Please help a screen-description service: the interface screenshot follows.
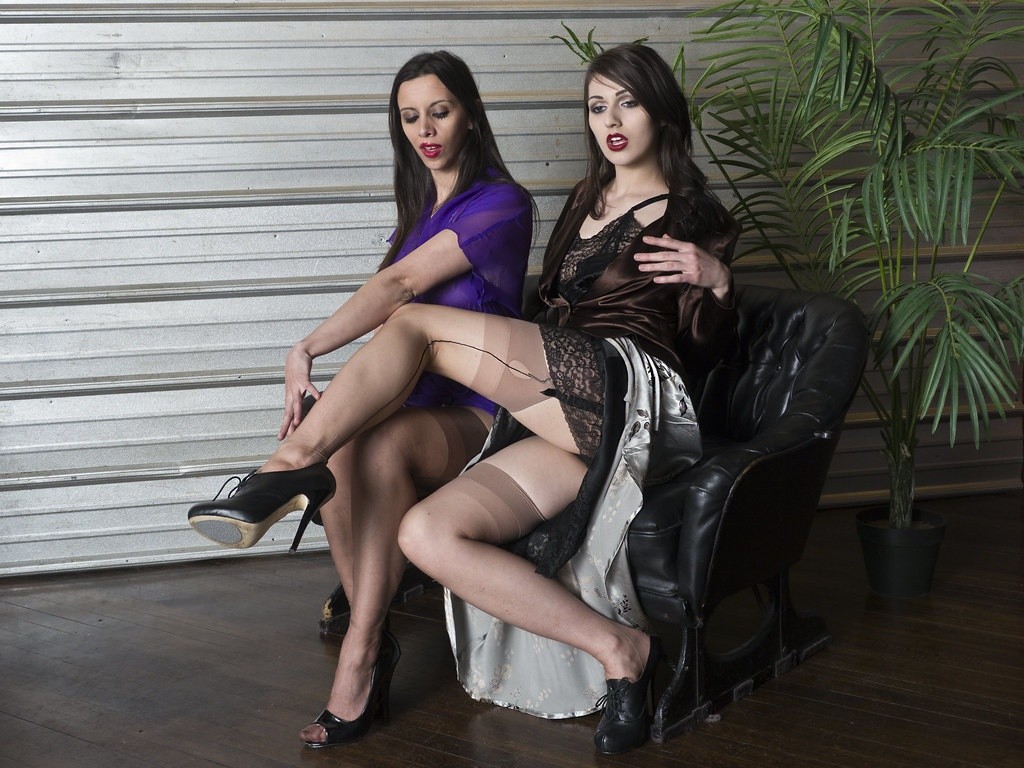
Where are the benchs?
[302,274,868,744]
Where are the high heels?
[300,630,401,749]
[594,634,663,754]
[188,463,337,555]
[317,582,350,644]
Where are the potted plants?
[548,0,1024,609]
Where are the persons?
[277,51,543,749]
[188,45,742,754]
